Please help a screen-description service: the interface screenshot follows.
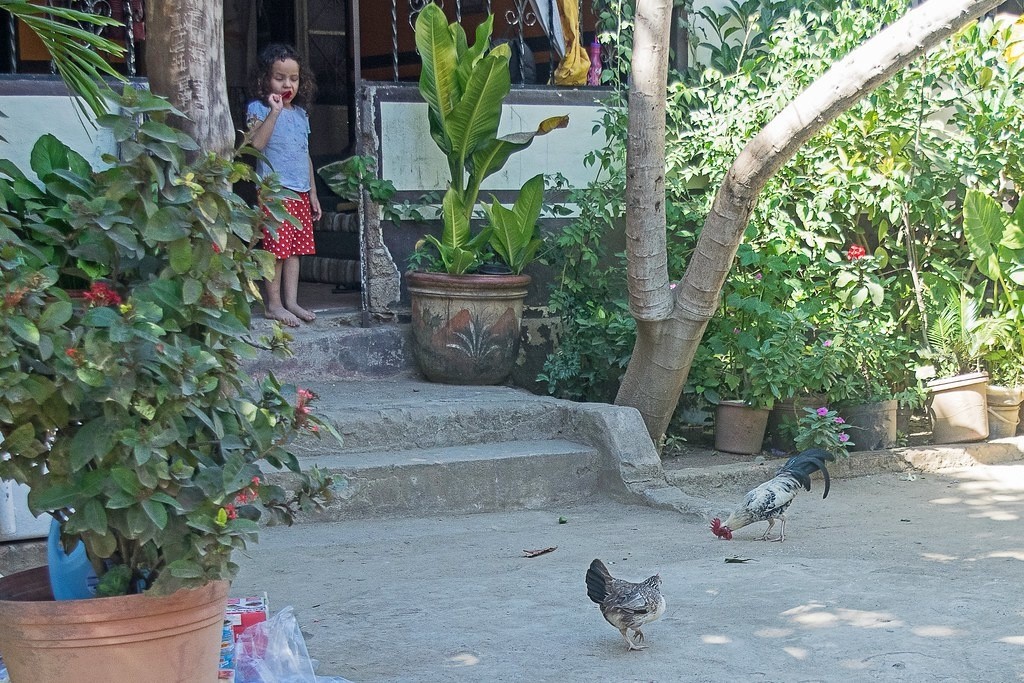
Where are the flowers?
[0,82,347,597]
[665,224,931,463]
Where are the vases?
[836,398,898,454]
[0,563,229,683]
[712,402,774,455]
[768,396,829,451]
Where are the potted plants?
[829,17,1024,443]
[407,0,571,386]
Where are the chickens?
[709,448,834,543]
[585,559,666,651]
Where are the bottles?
[220,619,237,683]
[587,42,602,86]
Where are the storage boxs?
[226,591,270,663]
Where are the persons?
[245,43,322,325]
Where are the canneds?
[218,618,239,680]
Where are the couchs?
[304,155,360,286]
